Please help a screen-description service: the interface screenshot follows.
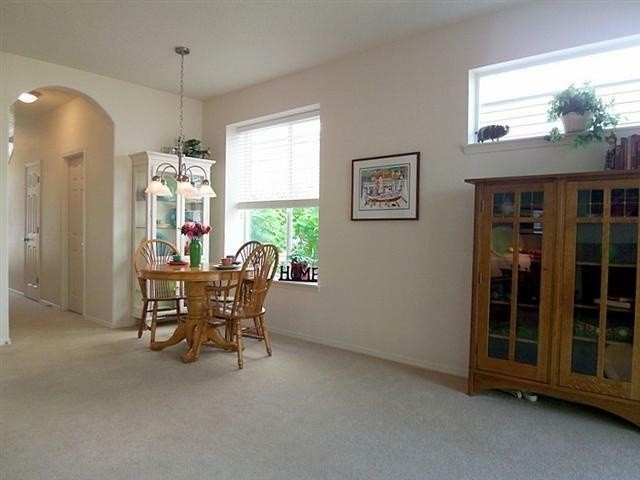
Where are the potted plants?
[544,76,624,149]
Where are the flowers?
[181,221,213,241]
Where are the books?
[593,296,632,310]
[607,295,633,302]
[614,134,640,172]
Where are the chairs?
[133,238,280,369]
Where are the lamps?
[144,47,217,200]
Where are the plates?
[167,261,188,265]
[218,264,240,268]
[215,265,237,270]
[491,225,524,256]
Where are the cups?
[226,256,236,263]
[172,255,182,262]
[221,258,233,266]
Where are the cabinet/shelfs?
[465,170,639,427]
[130,152,216,329]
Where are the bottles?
[184,241,189,255]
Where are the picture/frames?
[351,151,419,221]
